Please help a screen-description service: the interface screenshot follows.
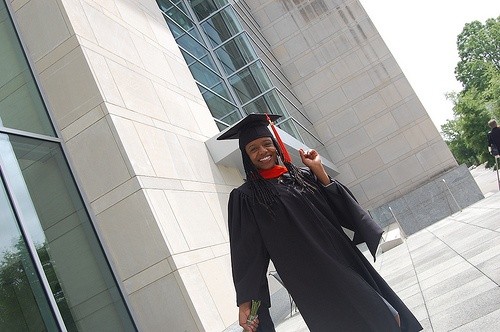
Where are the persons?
[226,122,426,332]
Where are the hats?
[216,113,283,149]
